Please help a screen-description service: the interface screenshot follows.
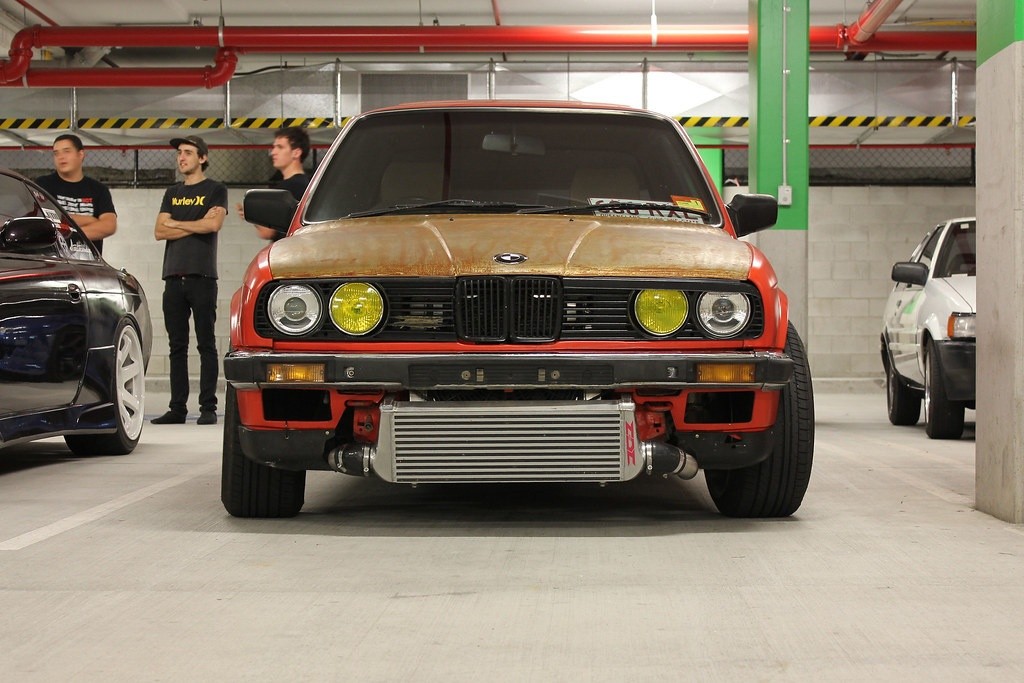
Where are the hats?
[170,135,208,155]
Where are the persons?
[237,126,310,243]
[150,136,228,424]
[34,135,117,258]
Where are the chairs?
[946,253,976,277]
[369,160,451,210]
[566,168,641,206]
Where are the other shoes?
[197,411,217,425]
[151,411,185,424]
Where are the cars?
[880,217,977,440]
[0,167,153,456]
[221,99,816,521]
